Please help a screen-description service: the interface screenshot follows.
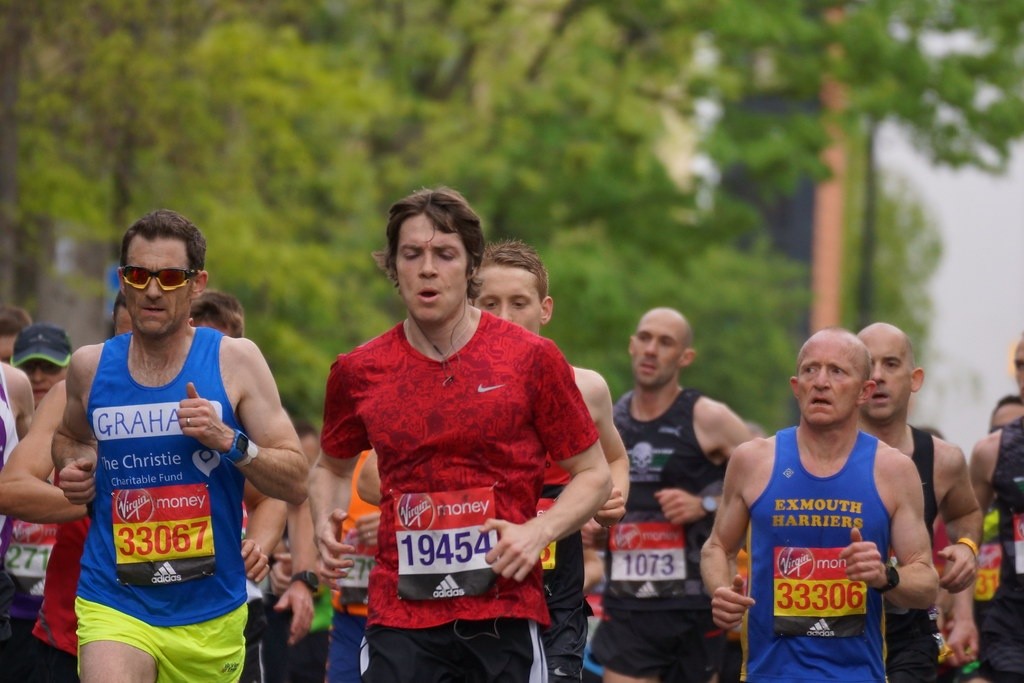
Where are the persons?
[700,328,939,682]
[310,187,614,683]
[0,291,1024,683]
[52,210,310,683]
[588,304,752,683]
[855,322,984,683]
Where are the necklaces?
[403,313,471,386]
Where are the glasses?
[122,265,196,291]
[1016,360,1023,367]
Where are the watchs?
[873,564,900,594]
[289,570,320,594]
[698,494,718,521]
[224,428,249,464]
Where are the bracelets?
[957,537,978,557]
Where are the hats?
[10,323,72,367]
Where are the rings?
[186,417,191,427]
